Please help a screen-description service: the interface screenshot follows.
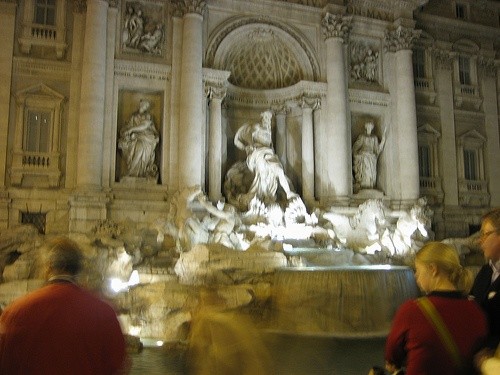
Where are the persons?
[0,188,440,297]
[117,100,161,177]
[232,111,298,199]
[349,41,382,81]
[385,360,404,375]
[468,211,500,374]
[385,242,485,375]
[122,5,172,53]
[185,307,270,375]
[0,237,130,375]
[368,366,384,375]
[352,121,388,190]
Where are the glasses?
[480,229,498,241]
